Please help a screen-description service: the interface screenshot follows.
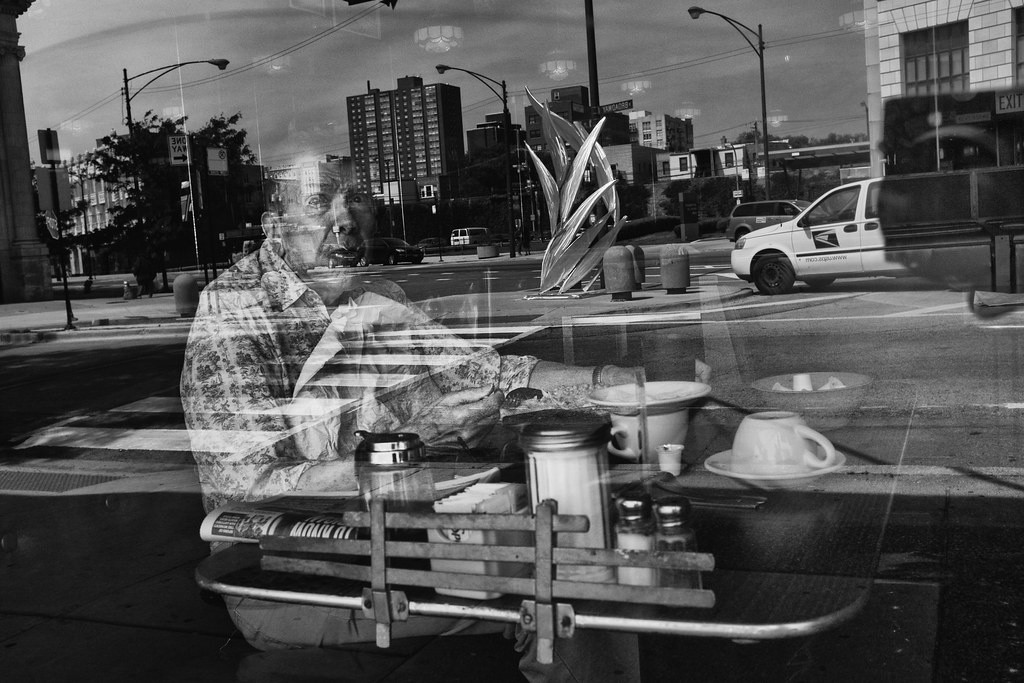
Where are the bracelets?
[590,359,608,388]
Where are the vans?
[725,198,831,243]
[450,227,490,246]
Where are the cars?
[319,244,356,269]
[357,238,424,267]
[414,237,447,250]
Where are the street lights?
[122,58,232,245]
[435,64,516,258]
[687,4,772,202]
[383,158,393,238]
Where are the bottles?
[651,495,705,596]
[614,489,656,595]
[518,415,616,592]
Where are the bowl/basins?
[750,367,880,436]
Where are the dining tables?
[193,337,922,676]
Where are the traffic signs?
[597,99,633,117]
[167,133,192,166]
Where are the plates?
[588,378,713,416]
[703,446,848,495]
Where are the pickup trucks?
[730,162,1024,296]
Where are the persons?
[183,122,657,682]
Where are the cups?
[653,443,685,479]
[455,449,499,464]
[607,413,693,465]
[729,408,835,476]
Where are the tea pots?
[349,426,441,517]
